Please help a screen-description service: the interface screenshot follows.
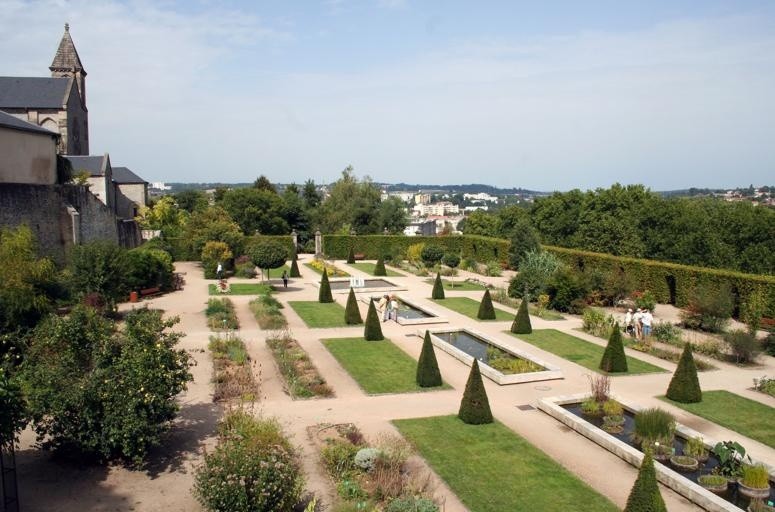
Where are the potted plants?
[736,463,771,501]
[670,456,698,472]
[697,474,728,493]
[607,404,626,426]
[711,440,752,484]
[682,435,709,463]
[607,425,624,436]
[649,444,675,461]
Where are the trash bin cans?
[130,292,137,301]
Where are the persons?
[625,308,633,336]
[639,307,654,338]
[216,262,224,281]
[281,270,290,288]
[377,293,388,323]
[632,308,642,340]
[388,294,401,322]
